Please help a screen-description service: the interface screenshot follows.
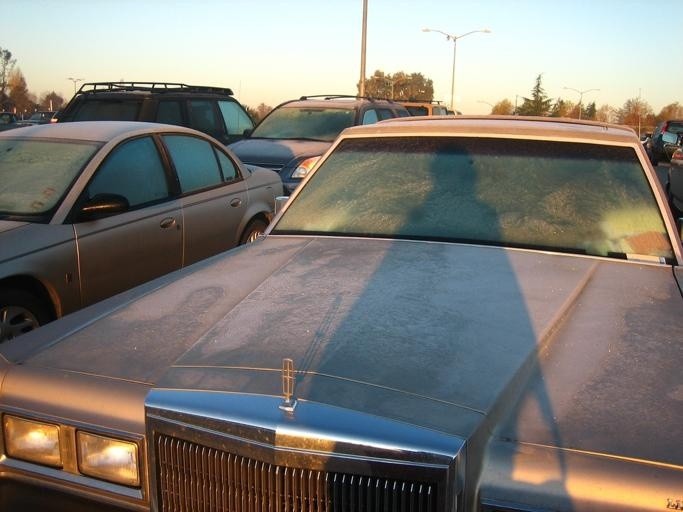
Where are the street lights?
[67,78,80,94]
[372,76,412,99]
[420,27,492,111]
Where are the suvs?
[0,114,683,512]
[392,98,447,117]
[56,82,256,144]
[225,93,411,201]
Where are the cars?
[638,119,683,222]
[446,110,463,115]
[0,120,283,346]
[0,111,62,132]
